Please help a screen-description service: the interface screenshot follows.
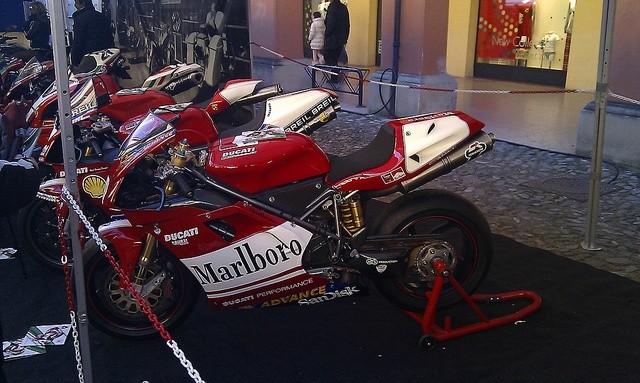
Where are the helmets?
[29,2,46,16]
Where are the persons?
[205,0,236,73]
[20,1,52,50]
[323,0,350,83]
[307,11,326,65]
[71,0,115,66]
